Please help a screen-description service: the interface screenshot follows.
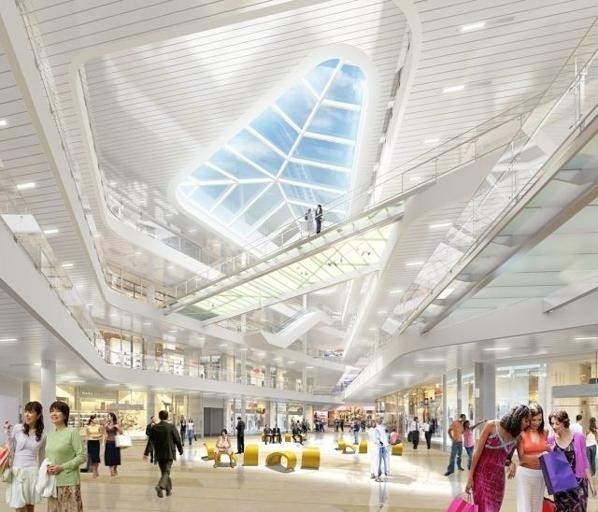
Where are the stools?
[203,434,403,469]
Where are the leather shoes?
[156,486,163,498]
[166,490,173,496]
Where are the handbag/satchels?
[447,492,479,512]
[539,448,580,493]
[586,433,597,447]
[0,438,14,482]
[114,430,133,448]
[544,497,558,511]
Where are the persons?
[4,401,47,512]
[584,417,597,478]
[314,204,323,234]
[422,417,432,450]
[512,399,552,512]
[569,415,583,435]
[545,408,588,512]
[461,419,486,471]
[303,208,315,239]
[444,414,465,477]
[44,401,87,512]
[410,416,420,450]
[103,412,123,477]
[464,405,532,512]
[142,410,400,499]
[84,415,104,478]
